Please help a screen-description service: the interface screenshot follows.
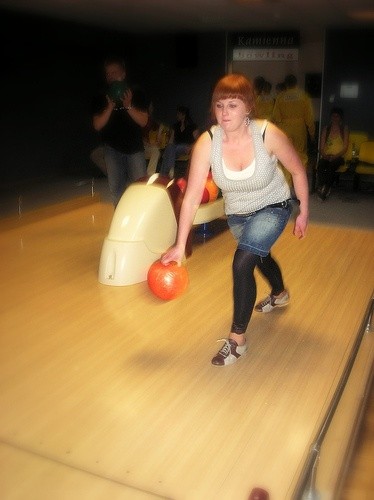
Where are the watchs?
[125,103,132,111]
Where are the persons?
[251,73,351,202]
[160,75,309,368]
[91,61,152,207]
[143,102,201,180]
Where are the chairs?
[333,131,374,193]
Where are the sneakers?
[254,289,290,312]
[211,335,248,367]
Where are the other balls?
[147,258,189,301]
[177,170,223,204]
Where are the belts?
[227,200,288,217]
